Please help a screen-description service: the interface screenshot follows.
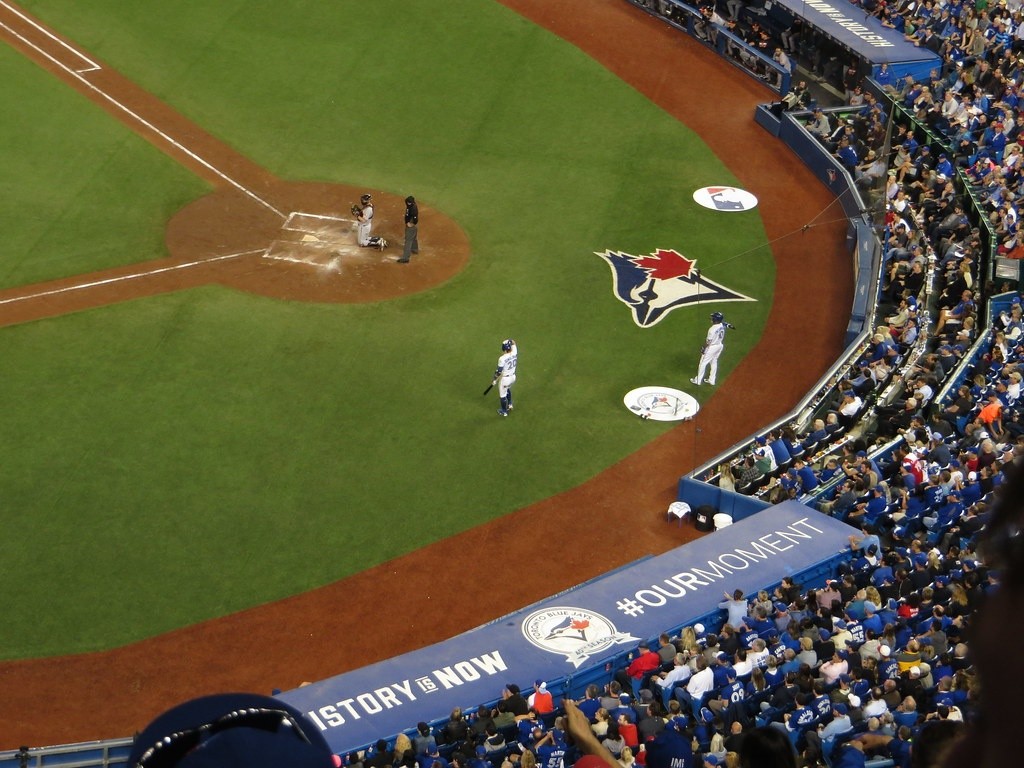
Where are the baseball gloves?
[350,204,359,217]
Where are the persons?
[492,339,518,416]
[634,0,1024,256]
[692,256,1023,522]
[351,194,389,252]
[396,196,419,263]
[332,522,1024,768]
[690,311,726,387]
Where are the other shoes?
[411,250,417,253]
[507,404,513,410]
[397,259,409,263]
[497,409,508,416]
[690,376,701,386]
[377,238,389,251]
[704,379,715,385]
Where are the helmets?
[710,311,723,322]
[361,194,371,205]
[502,340,511,351]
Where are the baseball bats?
[483,370,504,397]
[722,322,736,330]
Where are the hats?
[416,0,1024,768]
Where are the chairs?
[346,0,1024,768]
